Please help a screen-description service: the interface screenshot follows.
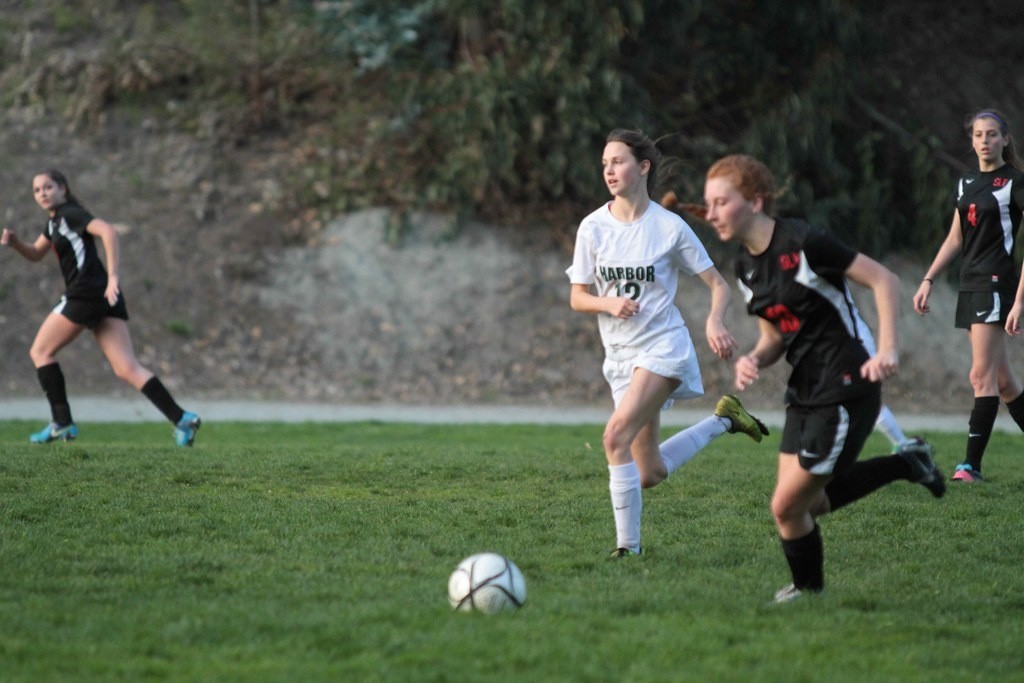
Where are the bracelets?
[922,278,934,284]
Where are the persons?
[0,170,200,448]
[565,126,771,562]
[911,109,1023,483]
[705,155,952,600]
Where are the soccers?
[445,552,528,617]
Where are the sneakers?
[891,442,903,455]
[902,436,948,499]
[174,412,200,447]
[774,582,801,602]
[607,549,644,557]
[28,422,77,442]
[951,464,982,483]
[715,394,770,443]
